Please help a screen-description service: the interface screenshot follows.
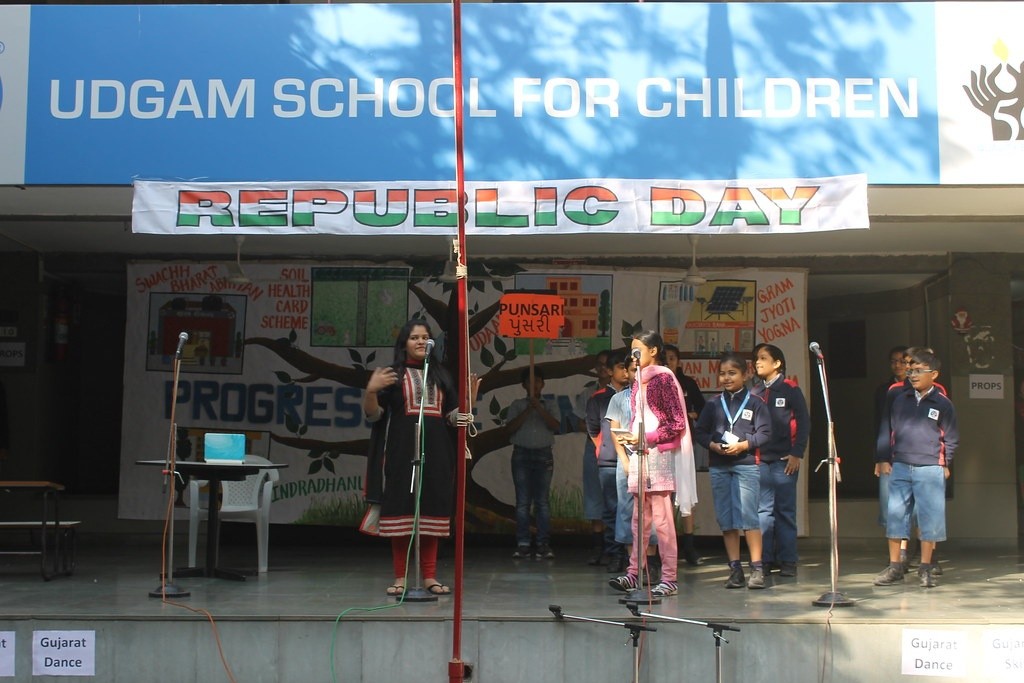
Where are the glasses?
[905,369,933,376]
[901,359,910,365]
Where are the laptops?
[205,433,245,465]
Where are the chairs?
[188,455,280,574]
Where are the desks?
[137,458,290,581]
[0,481,66,580]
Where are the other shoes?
[606,559,622,572]
[587,532,604,564]
[930,561,942,575]
[908,555,921,572]
[684,533,705,565]
[780,561,797,575]
[762,566,773,575]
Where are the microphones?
[424,338,435,359]
[176,333,188,359]
[810,342,824,360]
[632,349,642,359]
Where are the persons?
[869,346,958,586]
[574,344,706,586]
[506,370,561,559]
[610,330,688,593]
[693,355,772,592]
[362,320,483,597]
[749,344,812,576]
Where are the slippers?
[386,585,408,596]
[422,584,451,595]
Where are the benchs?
[0,520,81,578]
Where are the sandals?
[651,580,679,596]
[608,574,638,592]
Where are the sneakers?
[512,546,531,557]
[747,567,767,588]
[725,566,745,587]
[918,567,937,586]
[873,566,904,585]
[536,544,555,558]
